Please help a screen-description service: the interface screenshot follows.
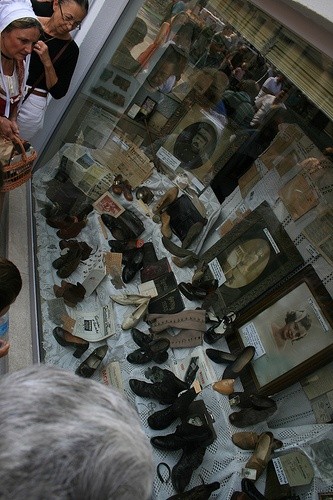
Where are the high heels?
[109,294,152,331]
[206,345,256,379]
[53,326,109,379]
[172,255,209,282]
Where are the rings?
[38,45,41,49]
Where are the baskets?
[0,135,38,193]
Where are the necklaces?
[44,18,60,47]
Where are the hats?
[197,128,211,142]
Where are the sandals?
[195,307,237,344]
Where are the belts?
[25,86,47,97]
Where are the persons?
[0,0,45,217]
[13,0,89,142]
[59,0,333,490]
[0,259,156,500]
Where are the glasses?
[60,4,81,31]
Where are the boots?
[147,307,207,350]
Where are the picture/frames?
[153,87,252,199]
[222,264,333,398]
[196,201,305,322]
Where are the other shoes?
[101,213,145,284]
[46,213,87,239]
[53,239,93,278]
[171,440,204,492]
[148,384,217,450]
[162,222,204,256]
[153,186,178,238]
[113,174,133,201]
[53,280,86,307]
[127,327,170,364]
[231,431,284,483]
[229,478,267,500]
[166,481,220,500]
[178,280,218,300]
[126,366,190,404]
[228,392,277,427]
